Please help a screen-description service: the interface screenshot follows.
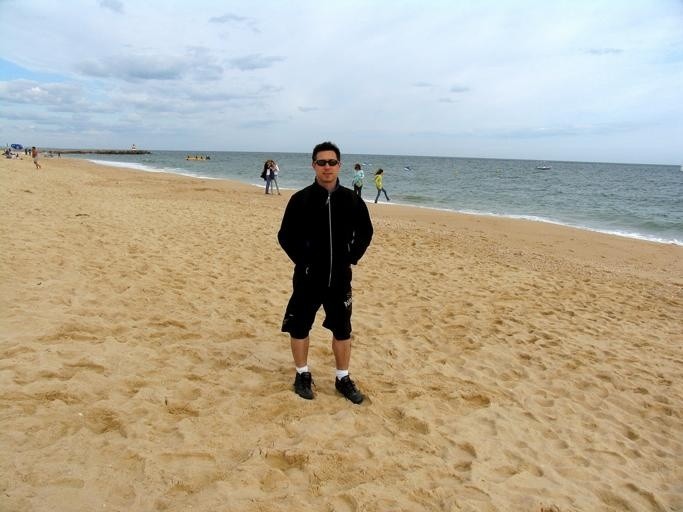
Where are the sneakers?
[335,375,363,404]
[294,371,316,399]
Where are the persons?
[262,160,282,194]
[278,142,375,403]
[373,168,390,204]
[351,163,364,198]
[3,143,58,169]
[186,154,210,161]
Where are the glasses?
[314,159,338,166]
